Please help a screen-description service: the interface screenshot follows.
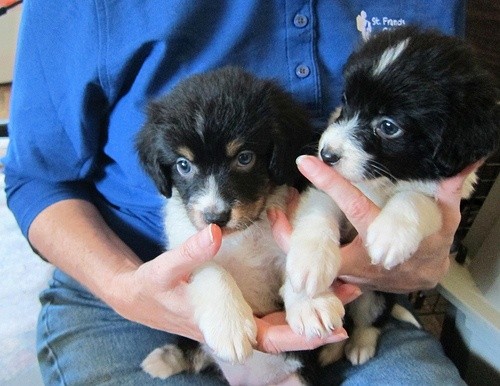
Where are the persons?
[1,1,474,386]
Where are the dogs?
[132,25,500,386]
